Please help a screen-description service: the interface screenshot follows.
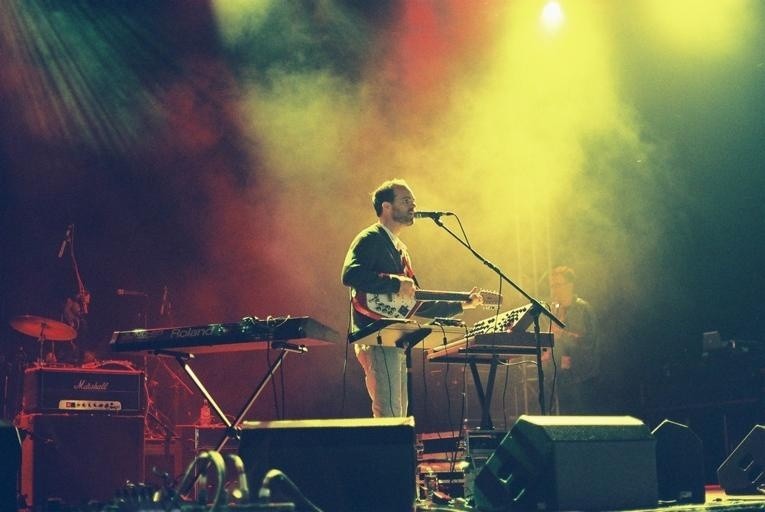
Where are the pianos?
[426,303,554,365]
[110,315,338,358]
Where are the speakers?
[234,414,417,512]
[651,419,711,502]
[473,414,659,512]
[717,424,765,494]
[19,412,147,509]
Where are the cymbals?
[9,315,78,341]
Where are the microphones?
[160,289,168,316]
[57,222,72,258]
[116,288,146,297]
[413,211,449,218]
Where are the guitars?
[351,273,503,321]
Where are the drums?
[81,360,154,422]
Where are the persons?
[341,176,486,419]
[542,265,596,416]
